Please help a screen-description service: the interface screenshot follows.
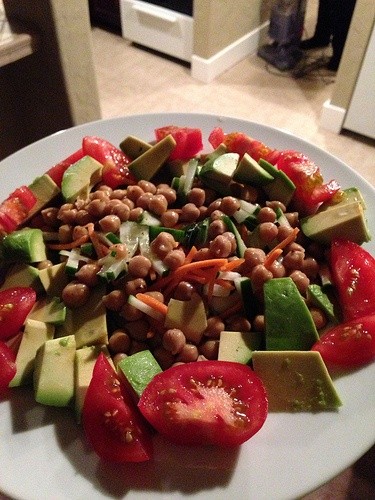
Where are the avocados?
[61,155,103,202]
[217,276,344,411]
[299,201,370,247]
[24,173,61,221]
[3,263,162,425]
[319,186,365,213]
[120,135,296,211]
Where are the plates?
[0,112,374,499]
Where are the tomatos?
[155,126,204,163]
[82,350,152,463]
[331,234,375,323]
[207,128,280,165]
[82,136,138,189]
[0,185,37,231]
[1,342,18,389]
[311,316,375,369]
[272,149,342,217]
[137,360,268,445]
[1,288,37,339]
[46,149,84,186]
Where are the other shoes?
[299,35,330,48]
[258,41,303,71]
[318,56,338,71]
